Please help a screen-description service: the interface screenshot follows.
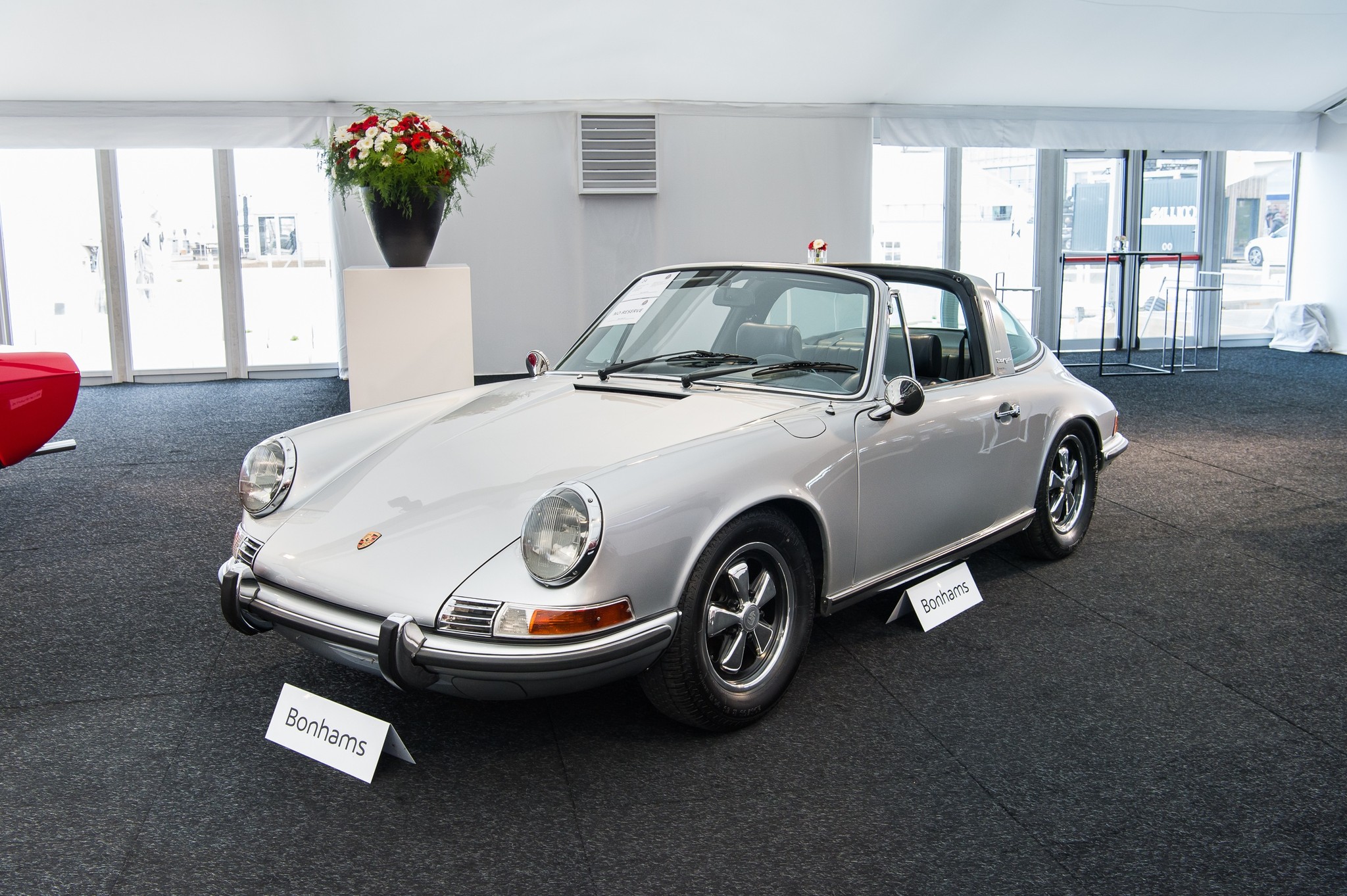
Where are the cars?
[1243,223,1289,267]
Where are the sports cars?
[212,256,1134,735]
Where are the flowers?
[303,103,495,226]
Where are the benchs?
[800,342,973,384]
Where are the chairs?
[721,323,801,367]
[840,331,945,398]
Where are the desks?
[343,263,475,413]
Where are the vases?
[359,186,445,267]
